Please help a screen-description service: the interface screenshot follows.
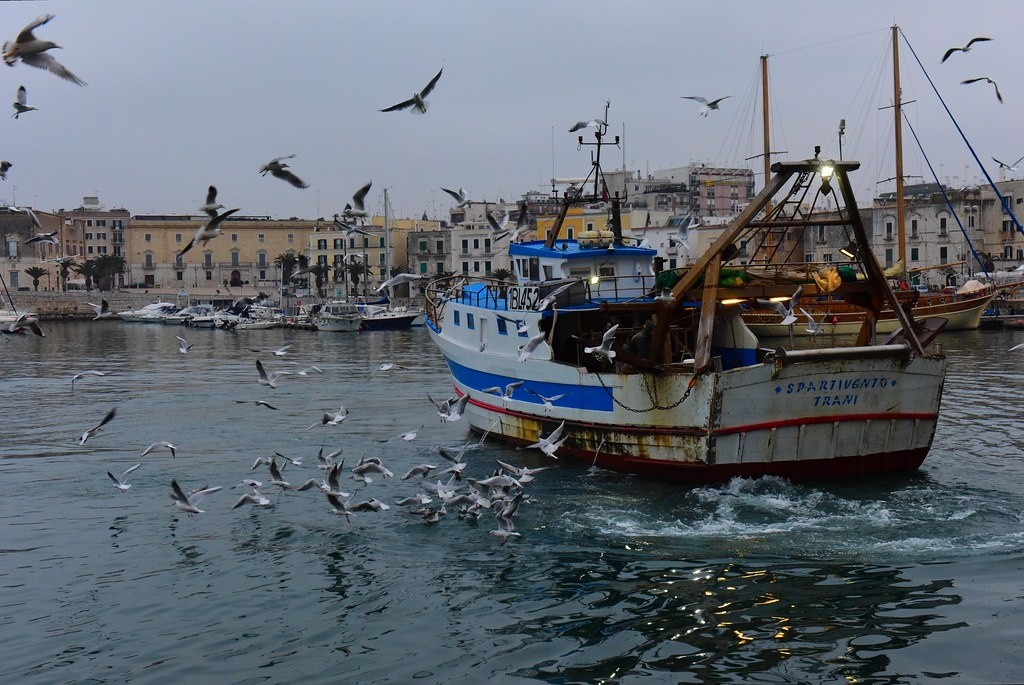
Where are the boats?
[418,145,948,489]
[0,271,40,334]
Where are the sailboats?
[113,183,432,333]
[682,19,1023,360]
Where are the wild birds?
[0,11,1024,543]
